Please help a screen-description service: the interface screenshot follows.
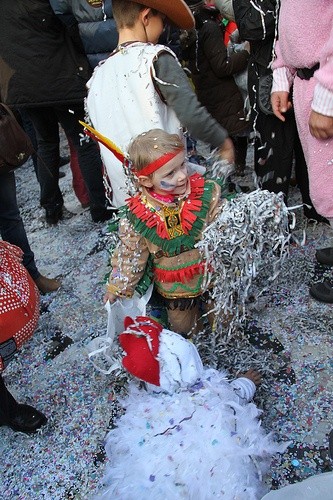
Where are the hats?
[131,0,195,31]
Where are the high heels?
[225,178,255,193]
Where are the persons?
[0,95,72,432]
[0,0,333,224]
[102,129,280,375]
[96,316,292,500]
[86,0,235,208]
[270,0,333,303]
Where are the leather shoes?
[0,404,47,431]
[35,278,61,296]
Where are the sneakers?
[309,281,333,303]
[316,248,333,267]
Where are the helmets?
[204,0,216,9]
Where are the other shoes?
[41,205,78,226]
[304,205,329,223]
[93,206,119,222]
[58,155,70,178]
